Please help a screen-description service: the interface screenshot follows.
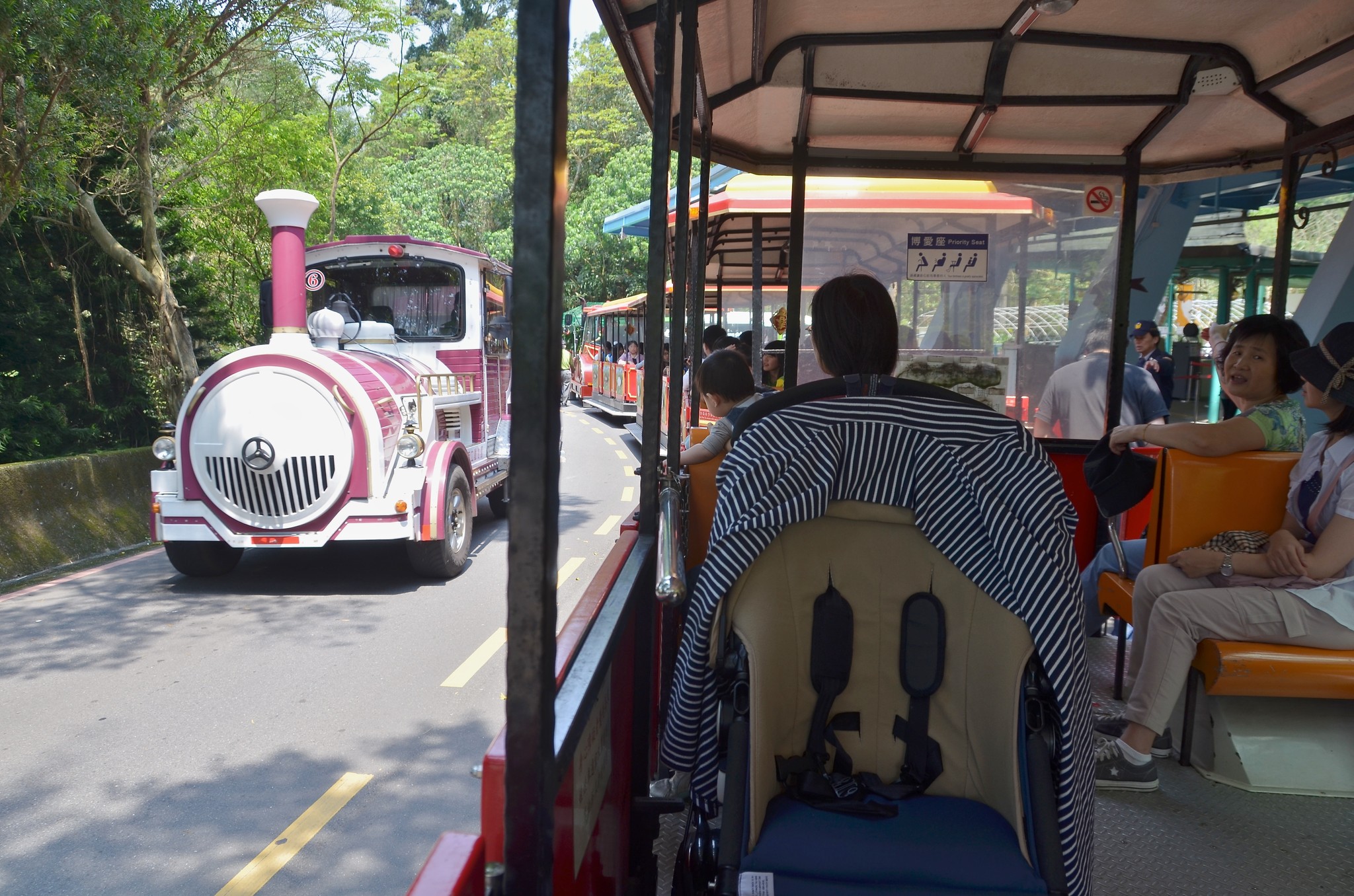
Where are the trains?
[400,1,1353,896]
[150,189,517,584]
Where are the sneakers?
[562,403,567,407]
[1092,713,1172,758]
[1093,737,1160,792]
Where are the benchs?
[1098,448,1353,768]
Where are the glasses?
[805,324,812,334]
[664,352,669,355]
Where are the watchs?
[1220,552,1235,576]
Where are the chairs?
[686,426,730,571]
[360,306,411,337]
[717,400,1068,896]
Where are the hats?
[1289,321,1354,408]
[1129,320,1158,337]
[1084,428,1157,518]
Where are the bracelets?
[1142,423,1151,445]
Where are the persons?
[439,291,461,336]
[1087,322,1354,792]
[1081,314,1310,642]
[661,349,781,475]
[706,274,898,453]
[1032,318,1170,448]
[1127,319,1174,426]
[560,324,919,407]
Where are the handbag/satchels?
[1205,539,1347,590]
